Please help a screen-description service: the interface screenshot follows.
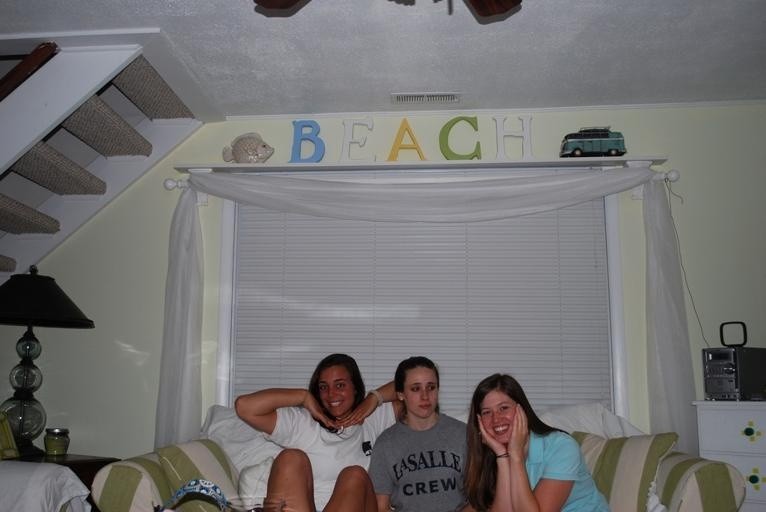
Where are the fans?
[254,1,522,18]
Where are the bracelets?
[496,452,508,458]
[368,390,384,405]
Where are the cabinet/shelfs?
[692,399,765,512]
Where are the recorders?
[702,347,766,401]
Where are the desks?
[6,454,121,512]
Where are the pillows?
[568,431,645,512]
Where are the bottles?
[44,427,70,456]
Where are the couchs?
[90,404,746,512]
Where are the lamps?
[1,268,93,455]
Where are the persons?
[233,353,405,510]
[461,371,609,512]
[366,356,477,510]
